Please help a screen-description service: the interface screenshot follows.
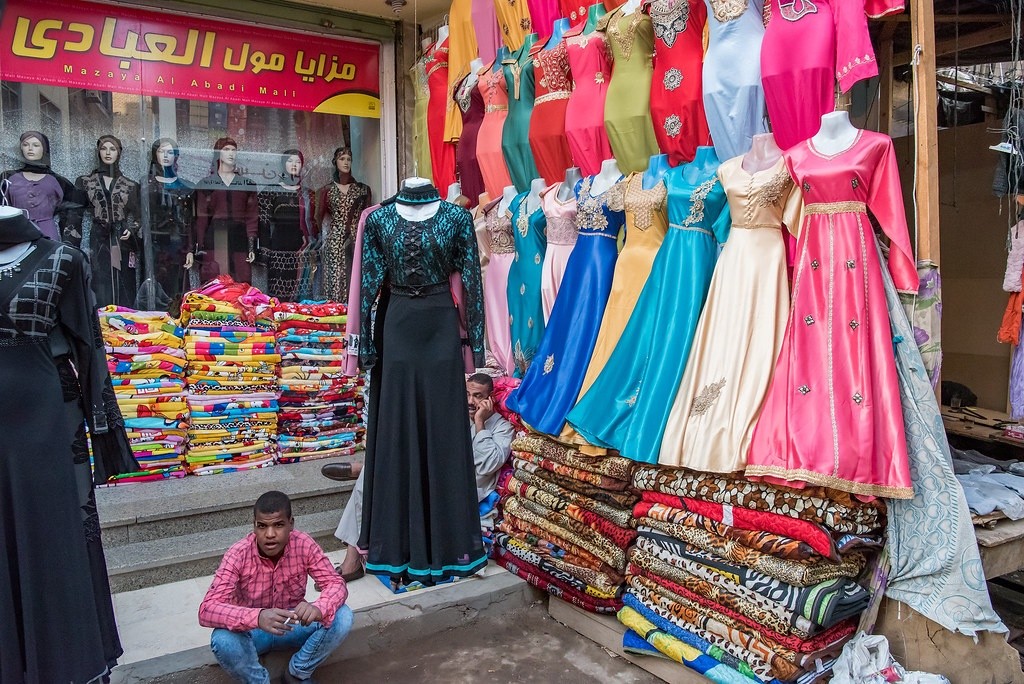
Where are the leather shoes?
[280,666,313,684]
[315,561,363,590]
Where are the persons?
[313,374,515,591]
[198,491,354,684]
[345,176,489,582]
[0,205,139,684]
[0,131,373,309]
[410,0,920,502]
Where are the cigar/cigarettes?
[283,617,291,625]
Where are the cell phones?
[485,391,497,405]
